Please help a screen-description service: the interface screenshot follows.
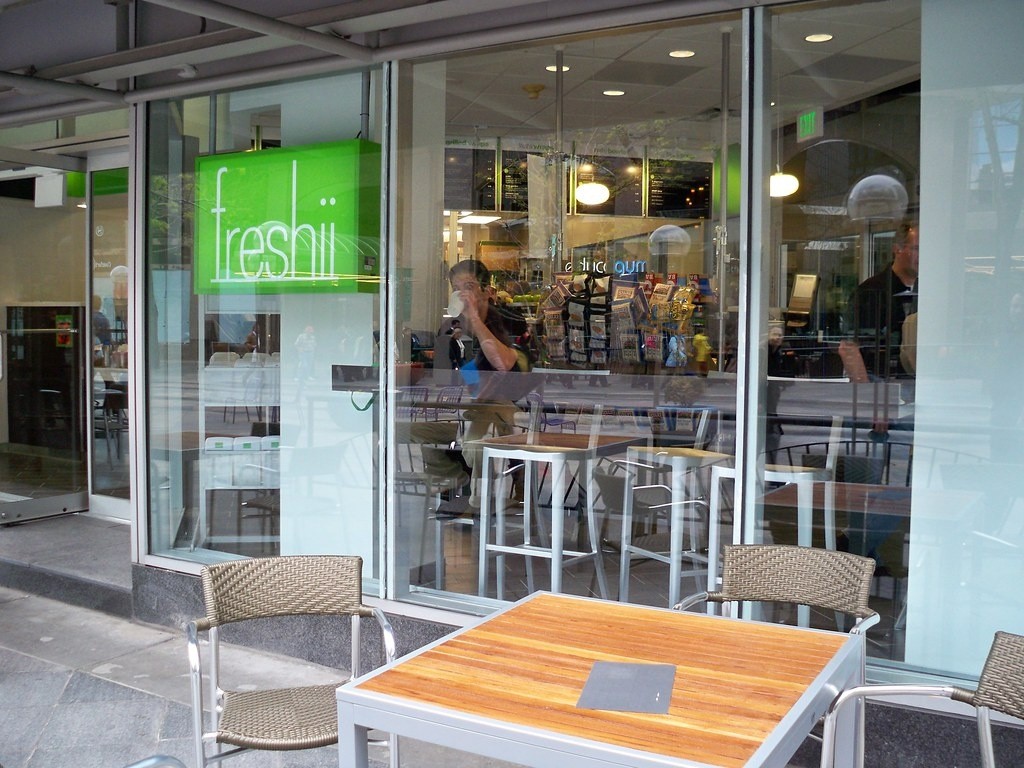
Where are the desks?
[754,480,912,635]
[334,589,867,768]
[465,431,647,600]
[334,379,914,431]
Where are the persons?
[415,259,539,518]
[838,222,920,435]
[90,295,784,473]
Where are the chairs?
[673,545,880,744]
[820,631,1024,768]
[235,491,278,557]
[187,555,401,768]
[398,369,913,665]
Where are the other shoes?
[435,495,472,519]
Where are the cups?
[447,291,465,317]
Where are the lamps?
[574,122,610,207]
[770,74,800,198]
